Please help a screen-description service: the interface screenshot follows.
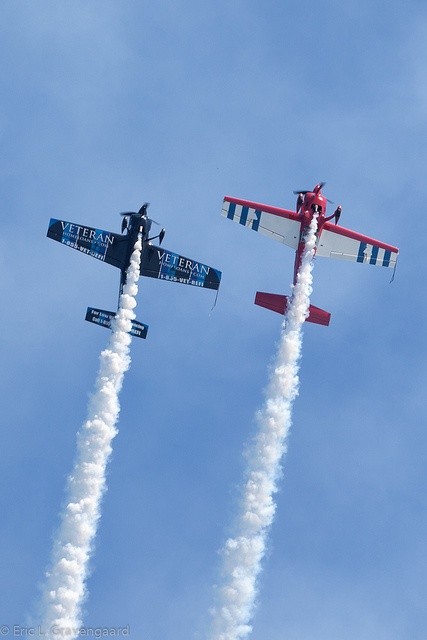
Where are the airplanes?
[46,202,222,340]
[221,183,399,326]
